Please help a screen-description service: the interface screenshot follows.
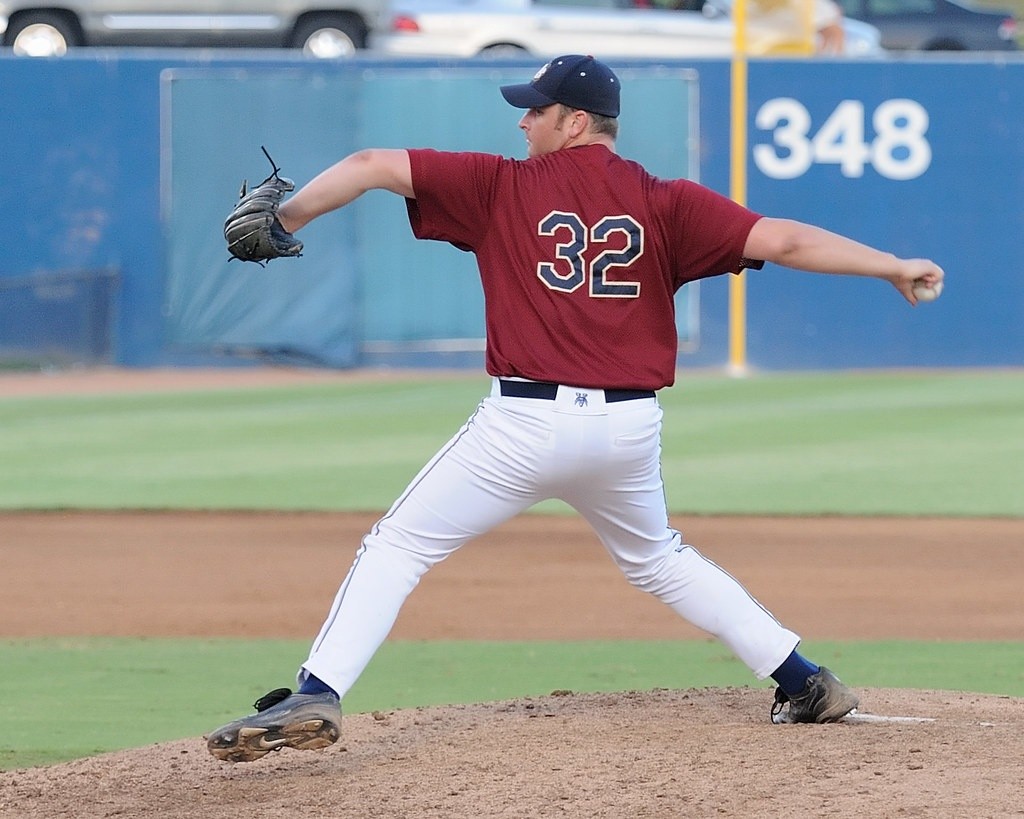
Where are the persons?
[204,55,945,763]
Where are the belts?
[498,378,656,403]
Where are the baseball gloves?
[224,144,306,266]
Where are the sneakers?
[209,687,344,763]
[769,666,863,727]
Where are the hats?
[503,57,623,120]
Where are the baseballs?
[912,276,943,303]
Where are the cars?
[840,0,1016,52]
[375,0,885,61]
[0,1,417,63]
